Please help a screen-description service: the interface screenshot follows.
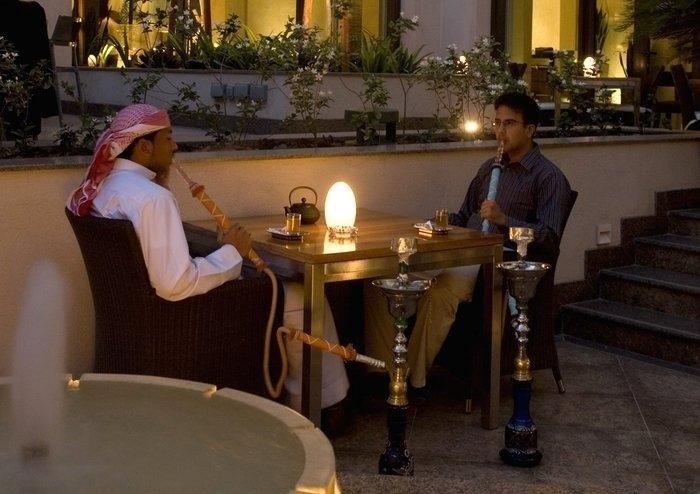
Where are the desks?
[548,75,644,132]
[182,205,506,431]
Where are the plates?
[413,223,452,235]
[266,226,313,241]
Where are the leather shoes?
[324,376,374,437]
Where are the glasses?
[494,118,529,129]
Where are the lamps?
[326,180,360,243]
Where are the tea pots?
[283,186,320,225]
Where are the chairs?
[425,188,580,416]
[670,65,700,131]
[491,63,526,84]
[60,203,281,395]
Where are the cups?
[435,208,448,230]
[286,213,301,235]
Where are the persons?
[64,102,354,432]
[364,88,569,410]
